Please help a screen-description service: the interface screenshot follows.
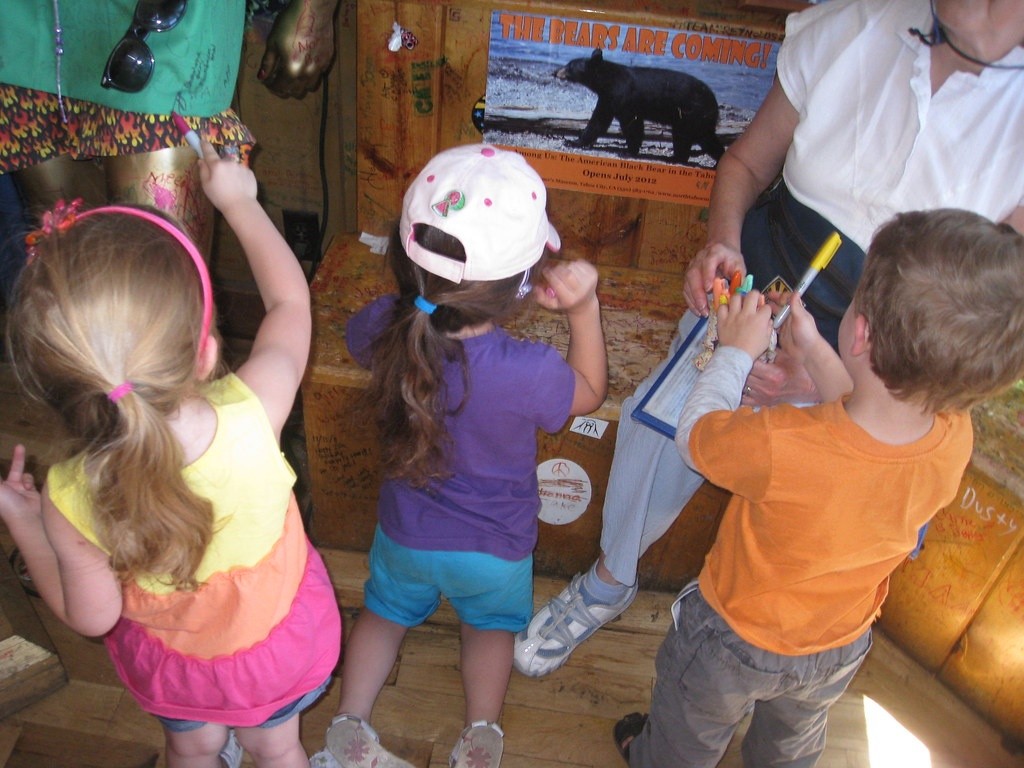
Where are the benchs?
[299,233,733,593]
[879,386,1024,747]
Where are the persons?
[0,139,342,768]
[0,1,338,266]
[512,0,1024,677]
[326,142,609,768]
[614,207,1024,768]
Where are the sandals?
[613,711,650,768]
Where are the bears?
[555,47,726,169]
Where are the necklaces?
[909,0,1024,70]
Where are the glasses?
[101,0,186,93]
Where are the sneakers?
[512,572,639,676]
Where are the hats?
[399,143,561,284]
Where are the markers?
[713,270,754,314]
[547,283,557,299]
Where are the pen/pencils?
[170,110,207,158]
[771,231,843,331]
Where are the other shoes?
[448,720,504,768]
[308,752,342,768]
[218,729,243,768]
[325,712,416,768]
